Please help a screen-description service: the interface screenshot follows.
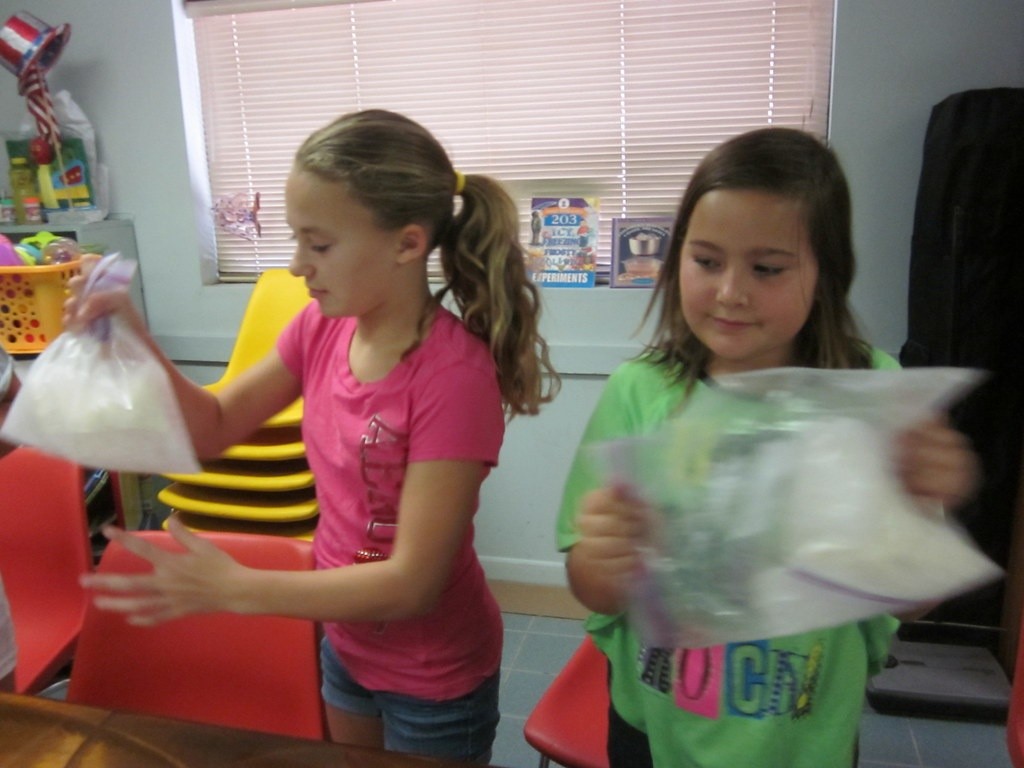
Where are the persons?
[61,108,563,768]
[554,128,975,768]
[0,349,35,692]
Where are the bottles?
[24,197,42,225]
[9,157,35,224]
[0,199,13,223]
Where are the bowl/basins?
[629,236,661,255]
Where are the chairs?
[0,269,610,768]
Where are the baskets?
[0,254,102,355]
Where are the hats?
[0,12,71,96]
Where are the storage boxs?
[7,138,93,221]
[0,253,104,355]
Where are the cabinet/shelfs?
[0,219,146,333]
